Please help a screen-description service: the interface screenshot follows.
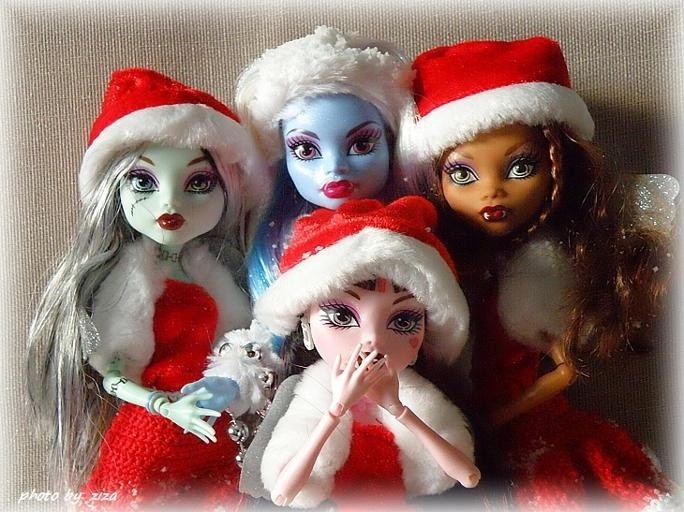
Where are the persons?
[22,25,684,512]
